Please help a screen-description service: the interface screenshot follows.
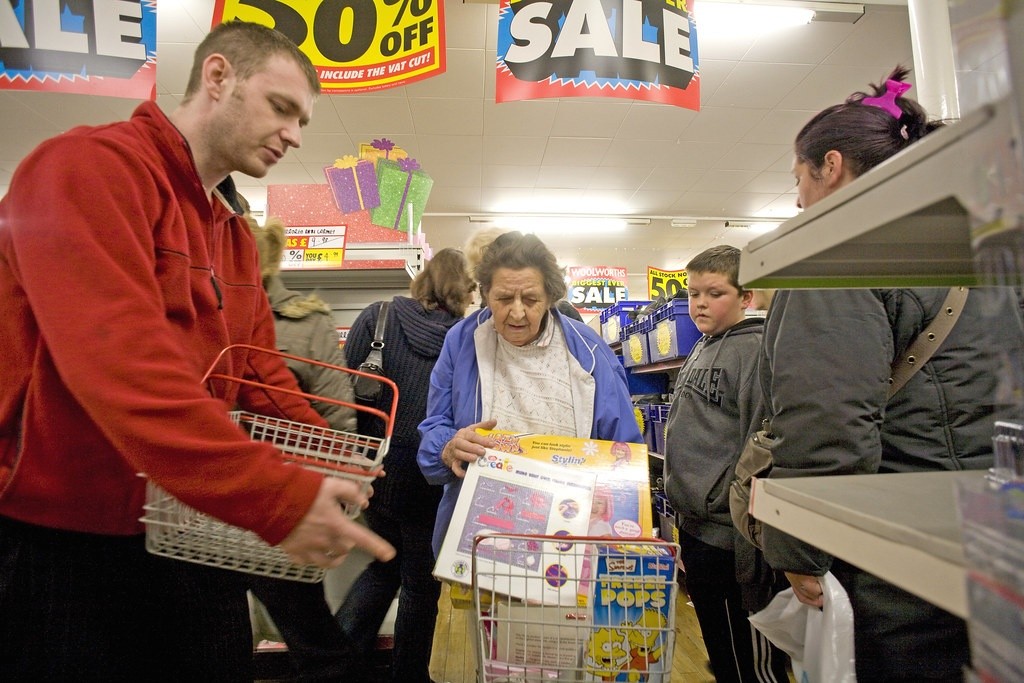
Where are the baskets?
[135,344,399,583]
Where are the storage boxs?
[586,284,710,545]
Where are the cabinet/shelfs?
[630,357,691,572]
[734,79,1024,623]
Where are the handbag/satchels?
[350,300,388,403]
[746,570,857,683]
[729,418,775,551]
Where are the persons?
[576,483,617,599]
[465,228,584,323]
[664,245,790,683]
[417,230,647,562]
[610,441,631,471]
[762,63,1024,683]
[0,20,397,683]
[334,246,476,683]
